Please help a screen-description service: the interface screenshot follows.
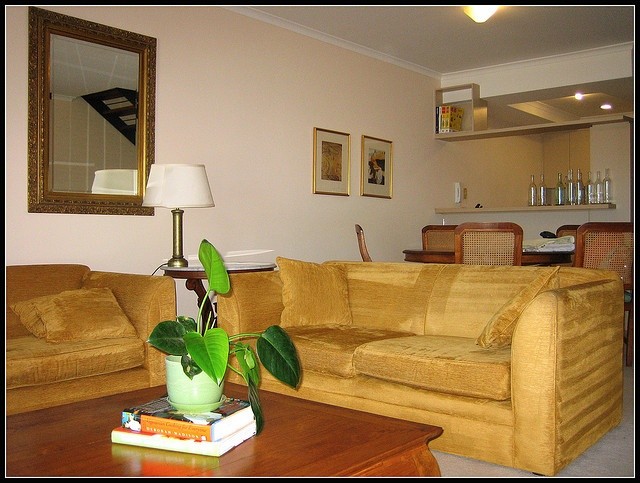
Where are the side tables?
[158,263,278,337]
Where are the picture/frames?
[312,126,352,195]
[361,132,394,198]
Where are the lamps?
[91,169,137,195]
[461,5,497,23]
[144,164,214,267]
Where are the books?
[111,419,257,457]
[121,397,254,442]
[436,105,464,134]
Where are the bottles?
[580,173,583,204]
[556,173,564,205]
[567,169,577,205]
[575,169,584,204]
[528,174,537,206]
[595,171,604,203]
[566,170,571,205]
[585,171,594,204]
[538,174,546,206]
[605,168,612,203]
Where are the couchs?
[4,265,177,414]
[210,261,623,476]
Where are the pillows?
[9,296,45,335]
[38,286,139,342]
[276,255,352,329]
[476,266,561,348]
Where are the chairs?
[454,221,522,266]
[573,221,633,366]
[354,225,374,262]
[422,224,459,252]
[555,225,580,244]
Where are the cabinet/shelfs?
[434,82,633,222]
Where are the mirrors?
[28,7,156,217]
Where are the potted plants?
[146,241,300,437]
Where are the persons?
[368,156,383,185]
